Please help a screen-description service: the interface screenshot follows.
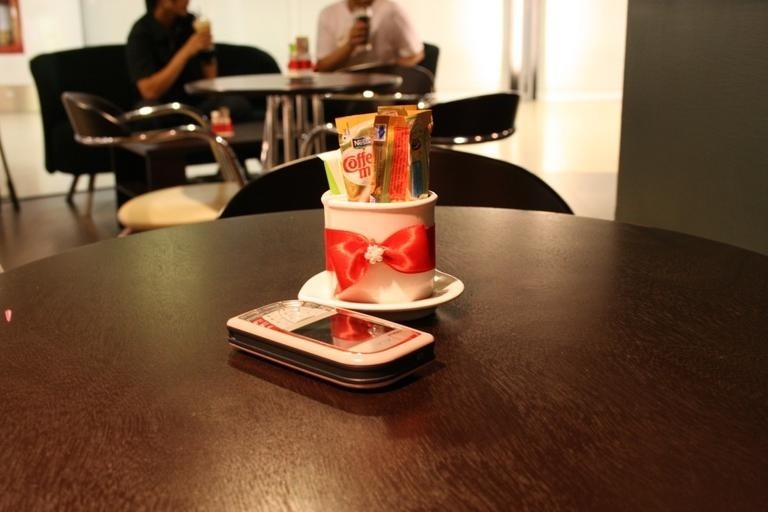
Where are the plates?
[298,267,466,322]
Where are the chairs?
[4,43,579,242]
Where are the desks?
[0,201,768,511]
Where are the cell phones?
[227,299,435,391]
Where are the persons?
[313,1,428,73]
[124,0,264,131]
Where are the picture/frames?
[0,0,25,57]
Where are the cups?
[288,53,312,79]
[210,117,235,139]
[354,15,373,52]
[194,20,209,32]
[319,188,438,303]
